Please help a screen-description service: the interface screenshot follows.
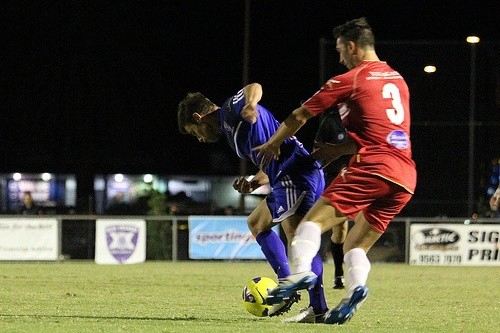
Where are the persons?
[178,83,331,324]
[21,194,43,215]
[251,19,417,325]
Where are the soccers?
[241,276,279,317]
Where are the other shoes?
[267,290,301,318]
[333,277,345,289]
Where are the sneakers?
[325,283,369,325]
[266,271,318,306]
[300,305,330,323]
